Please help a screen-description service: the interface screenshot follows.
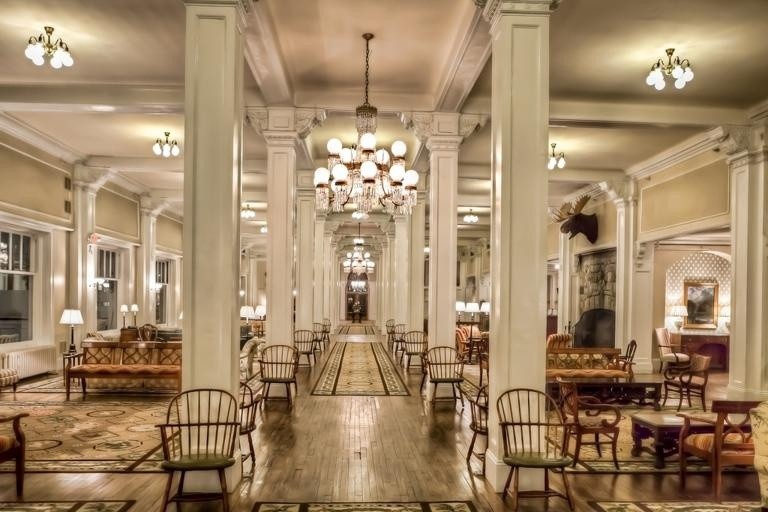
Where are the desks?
[628,411,752,470]
[63,353,83,386]
[670,333,730,373]
[547,353,609,370]
[545,373,664,411]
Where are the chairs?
[399,332,405,365]
[294,330,316,369]
[675,400,765,496]
[391,326,396,353]
[313,331,318,364]
[293,345,299,395]
[386,319,395,344]
[154,388,241,512]
[655,327,692,374]
[313,323,324,355]
[323,318,331,344]
[466,384,489,478]
[476,338,489,387]
[419,347,428,393]
[404,331,428,372]
[240,381,261,473]
[139,324,158,341]
[392,324,407,356]
[258,345,299,412]
[423,346,465,407]
[662,353,712,412]
[555,375,622,470]
[322,324,326,351]
[608,340,637,382]
[497,388,575,512]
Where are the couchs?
[546,347,636,377]
[462,326,484,358]
[151,333,182,341]
[750,402,768,509]
[547,333,572,349]
[64,342,182,401]
[85,377,178,393]
[456,328,482,363]
[239,338,266,383]
[481,332,489,353]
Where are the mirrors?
[683,282,720,329]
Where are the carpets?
[455,355,700,399]
[310,342,412,396]
[0,400,245,473]
[250,501,478,512]
[338,325,375,335]
[587,499,761,512]
[544,405,756,474]
[0,357,263,395]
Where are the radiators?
[1,345,58,379]
[103,334,120,342]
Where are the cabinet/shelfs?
[120,329,139,342]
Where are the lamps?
[255,305,266,336]
[350,273,366,289]
[120,304,129,328]
[463,209,479,223]
[240,204,256,218]
[645,48,695,91]
[313,33,419,216]
[152,132,180,157]
[178,312,183,320]
[547,143,565,169]
[342,222,375,273]
[59,309,85,352]
[669,306,688,332]
[464,302,480,364]
[352,210,370,221]
[130,304,140,327]
[25,26,74,69]
[240,305,256,338]
[260,225,267,233]
[480,301,491,332]
[456,301,465,328]
